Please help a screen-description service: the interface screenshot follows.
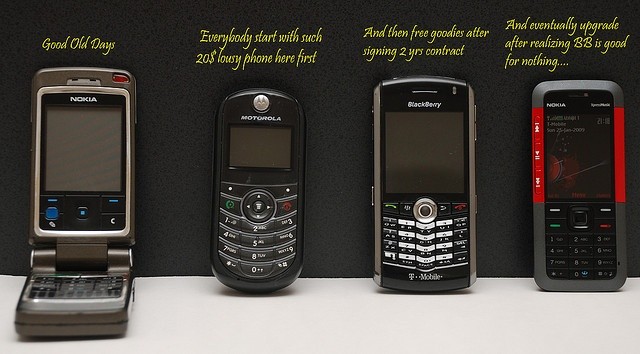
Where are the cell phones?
[530,79,627,291]
[371,71,479,291]
[14,62,137,338]
[208,85,308,291]
